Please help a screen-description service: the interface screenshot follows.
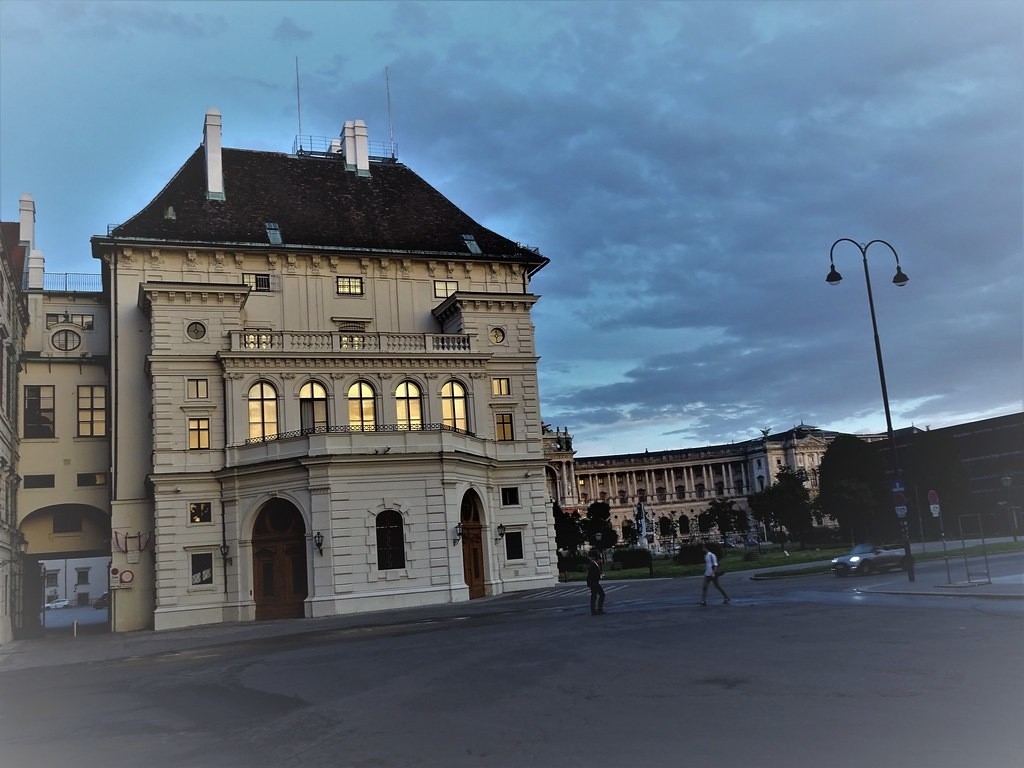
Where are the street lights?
[825,238,915,581]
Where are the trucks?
[93,592,109,611]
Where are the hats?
[595,552,602,560]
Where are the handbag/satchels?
[712,564,724,576]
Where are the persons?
[695,544,731,604]
[586,554,606,615]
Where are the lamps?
[313,531,324,557]
[220,544,233,566]
[494,524,506,545]
[453,523,465,546]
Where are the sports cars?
[829,542,907,578]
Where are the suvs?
[45,599,71,609]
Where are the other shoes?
[696,601,706,605]
[591,611,606,616]
[722,599,730,603]
